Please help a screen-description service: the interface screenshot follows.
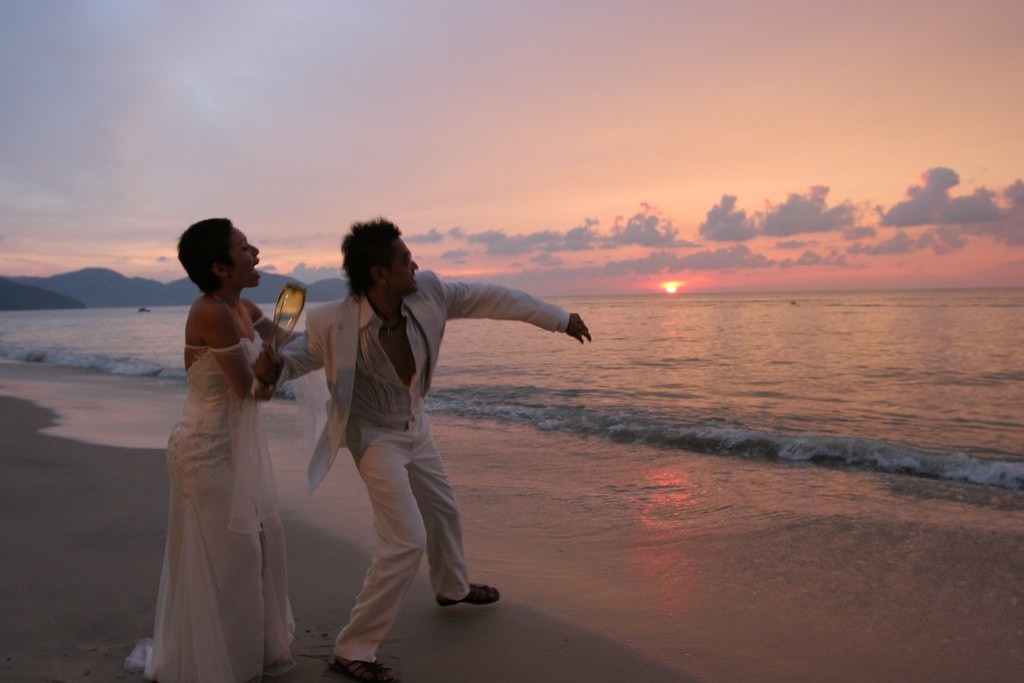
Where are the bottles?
[265,283,307,360]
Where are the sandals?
[333,655,399,683]
[436,582,500,608]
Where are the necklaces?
[379,315,401,338]
[208,290,242,316]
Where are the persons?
[165,217,308,682]
[253,218,592,682]
